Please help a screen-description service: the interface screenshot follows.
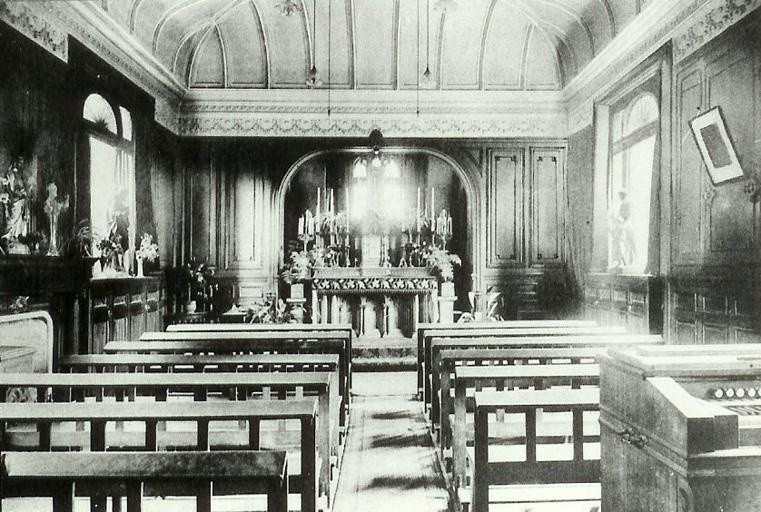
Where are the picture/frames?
[686,106,745,188]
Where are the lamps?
[421,0,435,84]
[371,146,381,167]
[306,0,323,88]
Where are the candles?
[297,185,350,235]
[400,185,454,236]
